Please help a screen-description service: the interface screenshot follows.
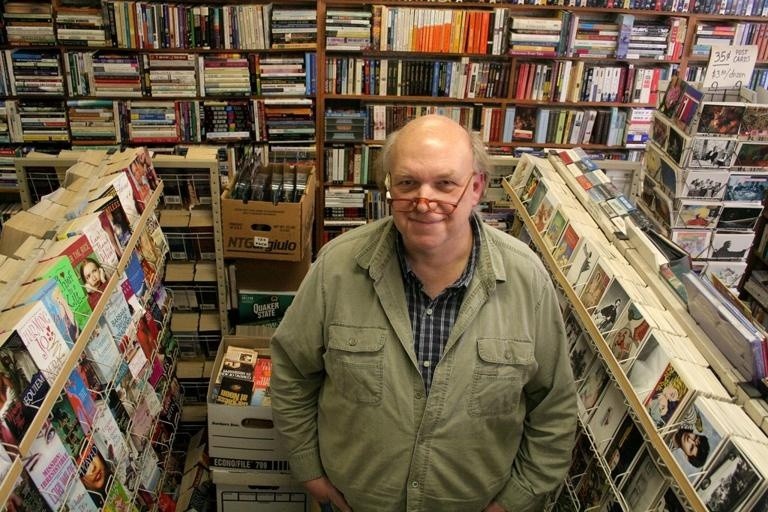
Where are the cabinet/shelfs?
[0,0,320,356]
[682,10,768,272]
[497,175,768,512]
[0,180,203,512]
[318,0,694,249]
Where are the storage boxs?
[215,483,312,512]
[220,160,315,263]
[206,337,287,463]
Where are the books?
[323,143,390,245]
[0,2,318,160]
[0,146,217,512]
[322,2,689,148]
[639,73,768,294]
[686,2,768,92]
[479,144,766,511]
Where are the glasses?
[380,167,478,220]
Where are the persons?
[269,114,578,511]
